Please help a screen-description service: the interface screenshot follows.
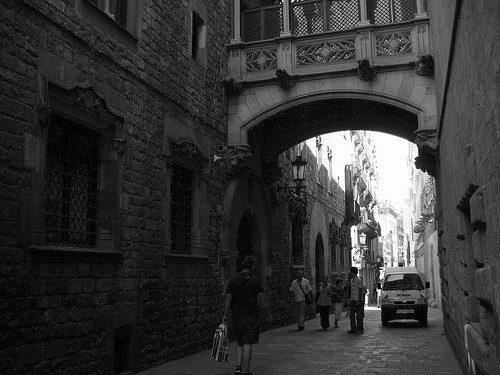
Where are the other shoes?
[347,330,363,334]
[235,365,252,375]
[298,327,304,330]
[334,323,340,327]
[323,327,327,330]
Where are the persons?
[289,270,314,330]
[223,255,272,375]
[376,282,383,308]
[317,267,367,334]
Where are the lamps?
[268,150,309,195]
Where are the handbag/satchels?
[305,292,314,306]
[211,322,231,362]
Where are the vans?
[377,267,430,327]
